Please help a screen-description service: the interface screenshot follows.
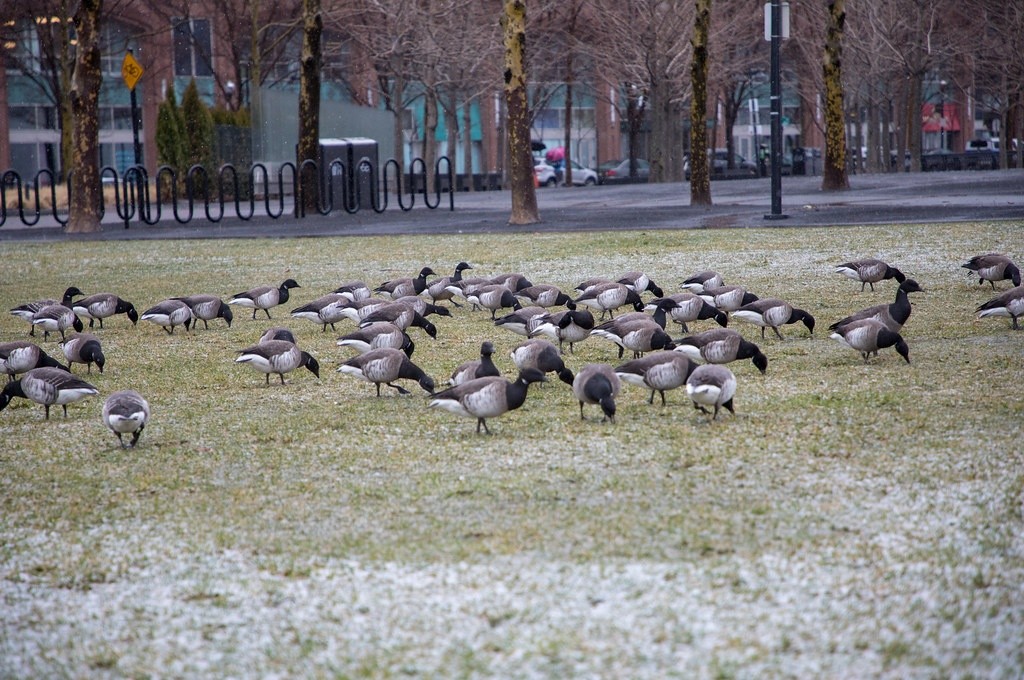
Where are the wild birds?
[423,367,550,435]
[613,351,701,406]
[491,273,533,295]
[0,367,99,419]
[57,333,106,374]
[572,364,622,424]
[651,293,728,333]
[10,286,84,337]
[466,285,522,321]
[444,279,493,312]
[258,328,296,345]
[102,389,150,448]
[670,329,768,375]
[73,293,138,329]
[169,295,233,330]
[355,303,438,339]
[617,271,663,311]
[335,324,414,360]
[493,305,550,339]
[573,277,644,321]
[518,285,576,311]
[446,341,500,387]
[30,304,83,343]
[828,319,911,365]
[419,262,474,308]
[373,266,436,300]
[227,279,301,320]
[677,272,725,295]
[335,300,391,324]
[396,296,453,318]
[686,363,737,420]
[961,253,1021,291]
[233,340,319,385]
[290,294,352,332]
[509,338,575,388]
[731,298,815,340]
[335,348,436,397]
[828,279,925,356]
[0,341,71,382]
[140,300,191,334]
[835,259,906,292]
[973,286,1024,330]
[334,281,371,302]
[695,286,760,317]
[588,296,683,359]
[530,299,594,354]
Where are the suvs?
[682,148,758,180]
[533,156,598,188]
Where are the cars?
[749,143,953,178]
[592,158,655,185]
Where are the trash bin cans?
[338,136,380,211]
[295,138,349,210]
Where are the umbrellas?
[531,140,565,162]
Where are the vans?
[964,137,1019,152]
